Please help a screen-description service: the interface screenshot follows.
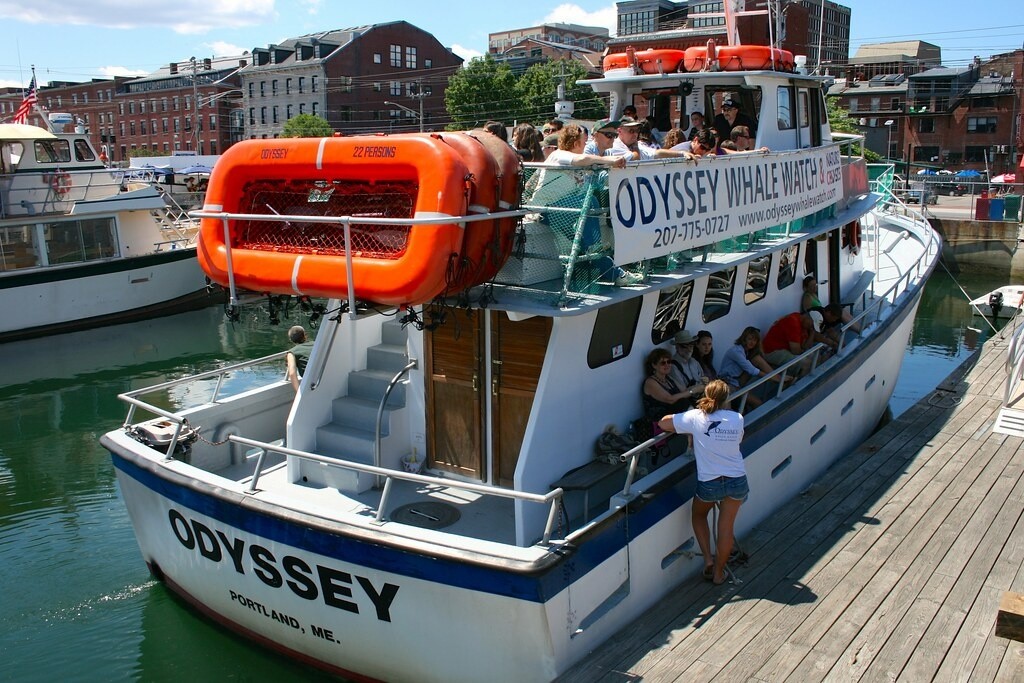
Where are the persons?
[659,380,750,584]
[481,98,771,284]
[287,326,314,391]
[644,276,861,428]
[187,177,209,201]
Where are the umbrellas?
[918,169,980,176]
[121,162,212,182]
[991,173,1015,183]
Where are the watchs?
[689,389,693,397]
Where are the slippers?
[703,566,715,579]
[713,567,728,584]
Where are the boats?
[969,283,1024,319]
[0,120,229,339]
[99,38,943,682]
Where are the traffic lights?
[1001,145,1010,154]
[990,145,999,154]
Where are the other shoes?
[782,377,797,388]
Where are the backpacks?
[633,417,672,456]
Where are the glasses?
[722,100,733,105]
[739,135,750,139]
[722,108,736,112]
[700,143,711,152]
[654,359,671,365]
[622,127,641,133]
[597,130,619,138]
[713,132,719,137]
[543,128,556,133]
[677,343,695,348]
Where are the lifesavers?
[849,221,862,255]
[52,169,72,193]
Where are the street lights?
[383,101,424,132]
[884,119,893,164]
[194,89,247,156]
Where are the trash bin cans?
[1004,195,1022,222]
[975,198,989,220]
[989,198,1004,220]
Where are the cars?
[887,174,918,189]
[935,182,968,196]
[904,187,938,204]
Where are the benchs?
[481,188,616,285]
[840,271,875,316]
[550,457,627,525]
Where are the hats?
[591,118,621,134]
[670,330,699,344]
[810,310,823,332]
[619,117,642,125]
[719,99,738,108]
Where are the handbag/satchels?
[597,430,649,464]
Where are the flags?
[14,76,38,124]
